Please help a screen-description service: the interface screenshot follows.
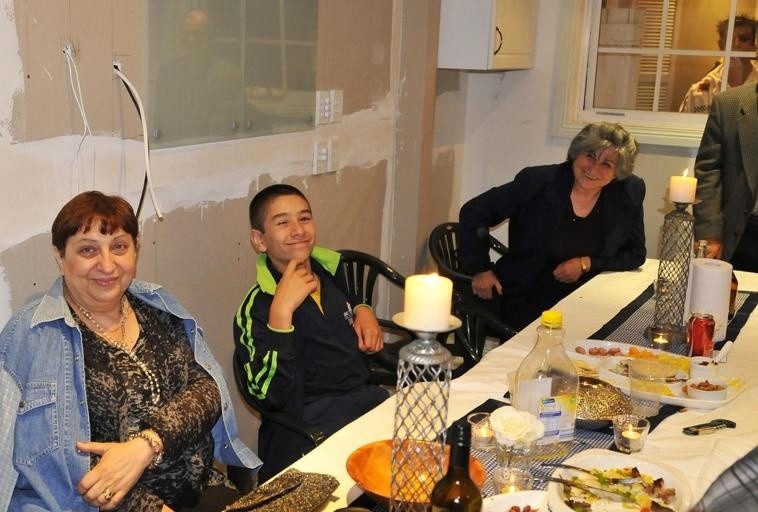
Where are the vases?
[492,468,533,494]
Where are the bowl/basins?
[343,438,484,507]
[535,373,635,431]
[687,378,729,403]
[692,355,721,381]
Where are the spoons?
[705,340,734,369]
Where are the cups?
[625,359,668,416]
[508,371,519,405]
[612,415,650,454]
[468,412,500,453]
[492,446,534,493]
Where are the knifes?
[533,476,637,506]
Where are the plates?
[547,449,691,512]
[562,337,664,372]
[477,492,567,512]
[590,357,729,412]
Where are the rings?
[103,488,114,501]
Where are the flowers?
[489,405,545,461]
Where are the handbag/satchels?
[226,468,340,512]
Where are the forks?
[541,461,643,486]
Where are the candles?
[669,171,699,205]
[404,272,453,332]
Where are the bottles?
[722,256,737,319]
[512,310,578,459]
[429,420,482,511]
[695,239,707,258]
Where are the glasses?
[731,35,751,46]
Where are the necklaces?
[69,292,161,404]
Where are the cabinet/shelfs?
[438,0,535,70]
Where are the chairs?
[428,221,523,356]
[329,248,481,392]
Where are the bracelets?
[579,257,588,274]
[126,431,164,471]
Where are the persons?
[679,13,758,114]
[458,121,646,340]
[232,184,392,476]
[691,79,757,275]
[1,190,265,511]
